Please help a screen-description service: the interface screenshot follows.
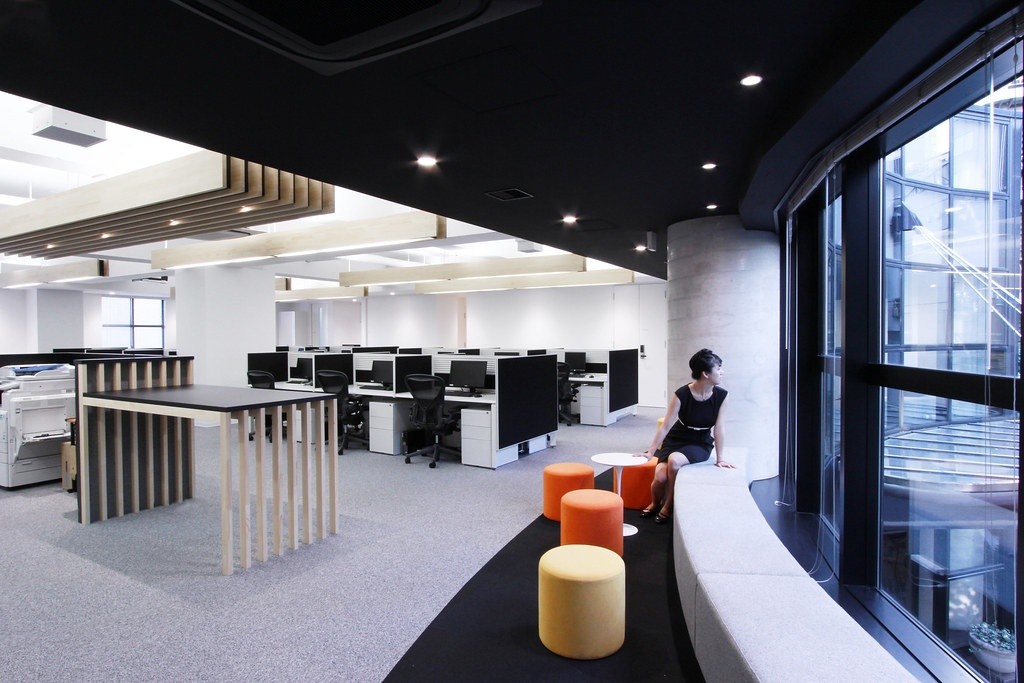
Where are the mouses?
[286,381,308,384]
[589,376,594,378]
[474,394,482,397]
[387,387,393,391]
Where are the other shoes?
[639,506,656,518]
[654,511,671,524]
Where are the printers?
[0,364,76,488]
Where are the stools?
[613,456,659,508]
[538,544,625,659]
[542,462,594,521]
[560,489,624,559]
[657,418,665,450]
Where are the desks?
[76,355,339,574]
[556,348,640,427]
[591,453,649,537]
[249,353,550,471]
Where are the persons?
[632,346,738,526]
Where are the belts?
[679,418,710,431]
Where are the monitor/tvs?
[565,352,586,374]
[295,346,547,392]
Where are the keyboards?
[359,385,387,390]
[445,390,475,397]
[569,374,585,378]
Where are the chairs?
[247,370,288,443]
[405,374,460,468]
[316,370,370,455]
[557,362,580,426]
